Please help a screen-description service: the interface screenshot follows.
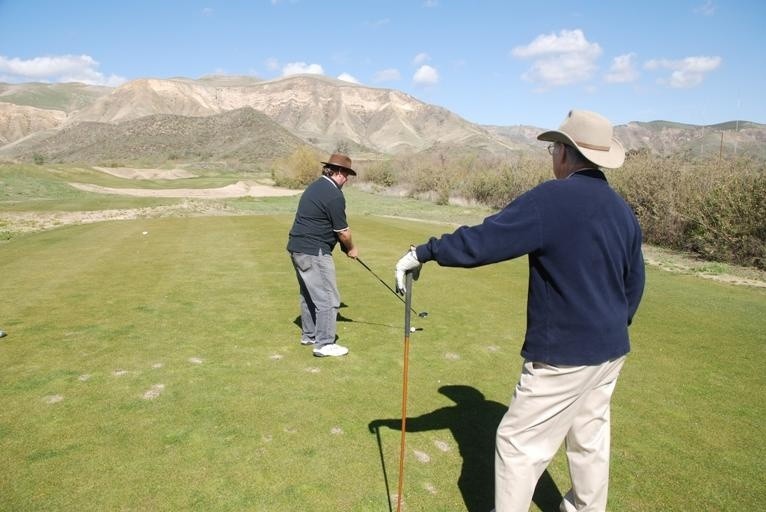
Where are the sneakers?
[312,342,349,357]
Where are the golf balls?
[410,327,416,333]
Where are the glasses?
[546,142,570,156]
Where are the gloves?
[395,244,422,296]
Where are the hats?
[536,110,625,169]
[321,153,356,176]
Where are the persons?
[391,107,649,512]
[284,153,361,358]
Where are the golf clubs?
[352,257,428,317]
